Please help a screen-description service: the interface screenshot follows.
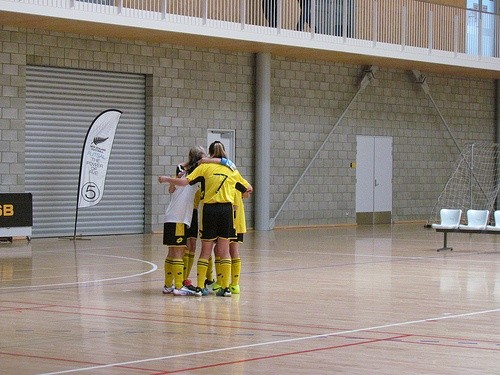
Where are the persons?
[182,145,215,290]
[210,188,246,294]
[158,141,253,297]
[163,162,201,296]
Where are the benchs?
[431,209,500,252]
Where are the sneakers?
[162,279,242,296]
